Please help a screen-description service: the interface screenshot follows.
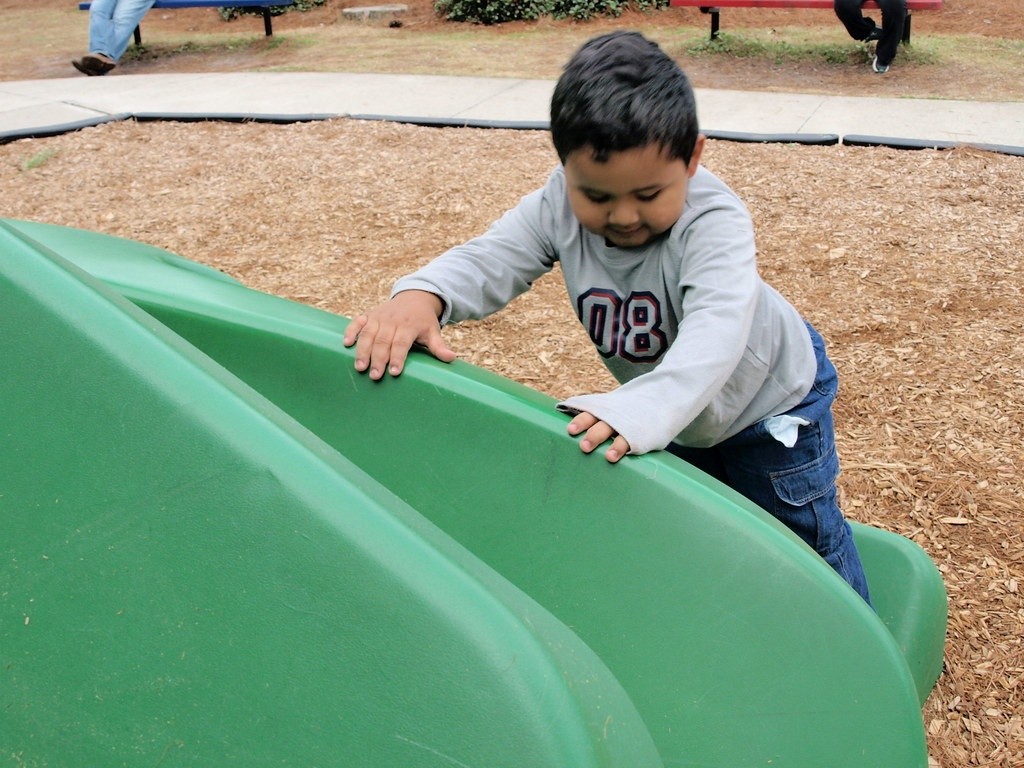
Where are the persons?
[71,0,155,77]
[343,29,876,613]
[834,0,908,73]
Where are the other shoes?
[865,23,882,43]
[872,45,889,73]
[82,54,116,74]
[72,59,105,76]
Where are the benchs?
[670,0,942,45]
[79,0,294,45]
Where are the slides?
[0,214,951,768]
